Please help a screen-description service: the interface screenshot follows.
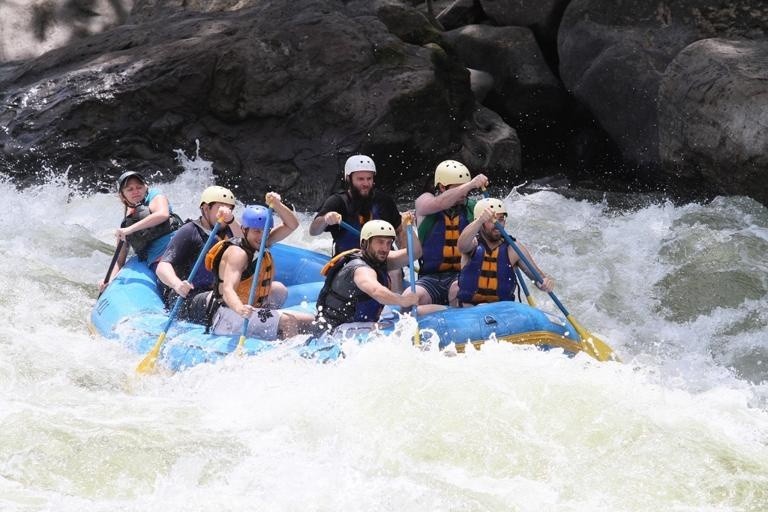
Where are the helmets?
[240,204,275,230]
[359,219,397,248]
[345,155,377,182]
[198,185,236,212]
[117,170,146,193]
[433,159,472,189]
[473,197,509,221]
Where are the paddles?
[494,220,621,364]
[137,216,229,376]
[226,203,275,368]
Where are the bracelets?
[224,217,234,225]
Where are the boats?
[91,237,620,378]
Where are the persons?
[310,155,404,292]
[210,192,313,345]
[457,198,553,306]
[315,212,423,336]
[155,183,288,323]
[98,172,184,291]
[402,159,489,315]
